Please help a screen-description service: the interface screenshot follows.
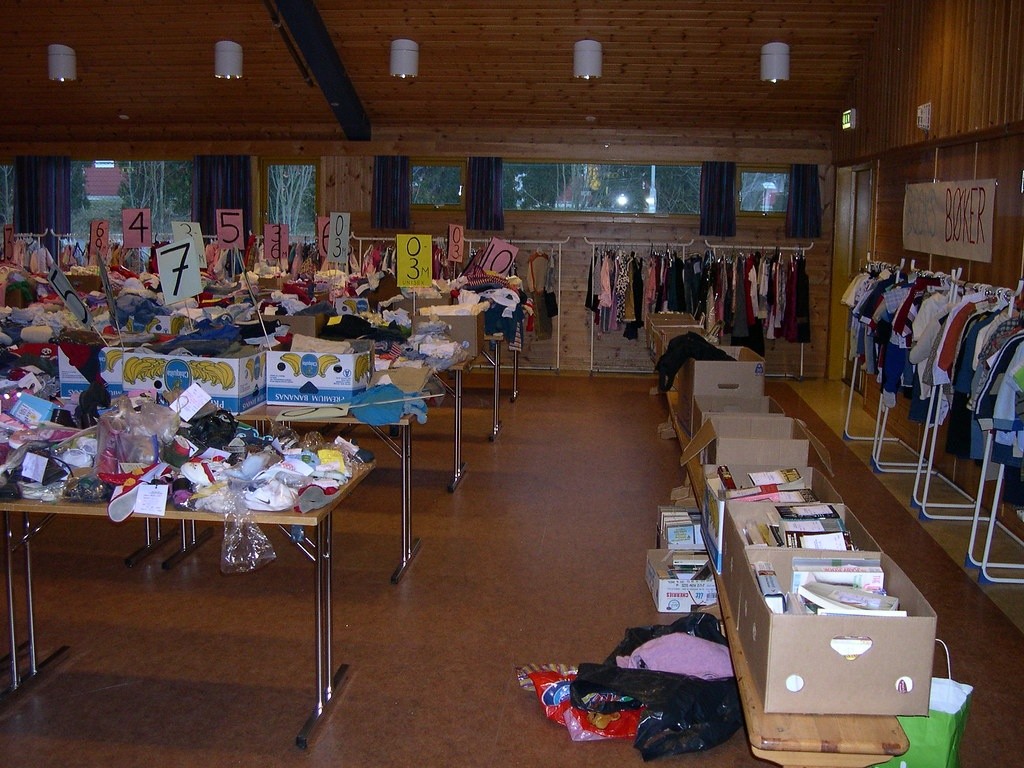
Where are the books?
[655,468,912,695]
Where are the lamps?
[759,41,790,86]
[48,45,77,83]
[572,40,602,80]
[212,40,244,81]
[390,39,419,79]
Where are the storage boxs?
[57,345,140,406]
[413,306,487,357]
[645,313,695,350]
[258,309,327,334]
[66,273,102,293]
[649,326,719,365]
[718,504,887,631]
[259,273,295,289]
[644,549,719,612]
[122,351,267,416]
[678,418,835,478]
[212,293,257,308]
[675,346,768,434]
[737,548,938,718]
[3,287,29,310]
[113,313,192,337]
[392,292,452,320]
[265,347,374,406]
[656,471,707,549]
[694,395,785,440]
[333,296,371,317]
[701,464,845,575]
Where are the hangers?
[12,232,808,276]
[858,259,1024,319]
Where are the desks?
[0,441,378,754]
[160,346,433,586]
[280,323,479,496]
[388,331,504,443]
